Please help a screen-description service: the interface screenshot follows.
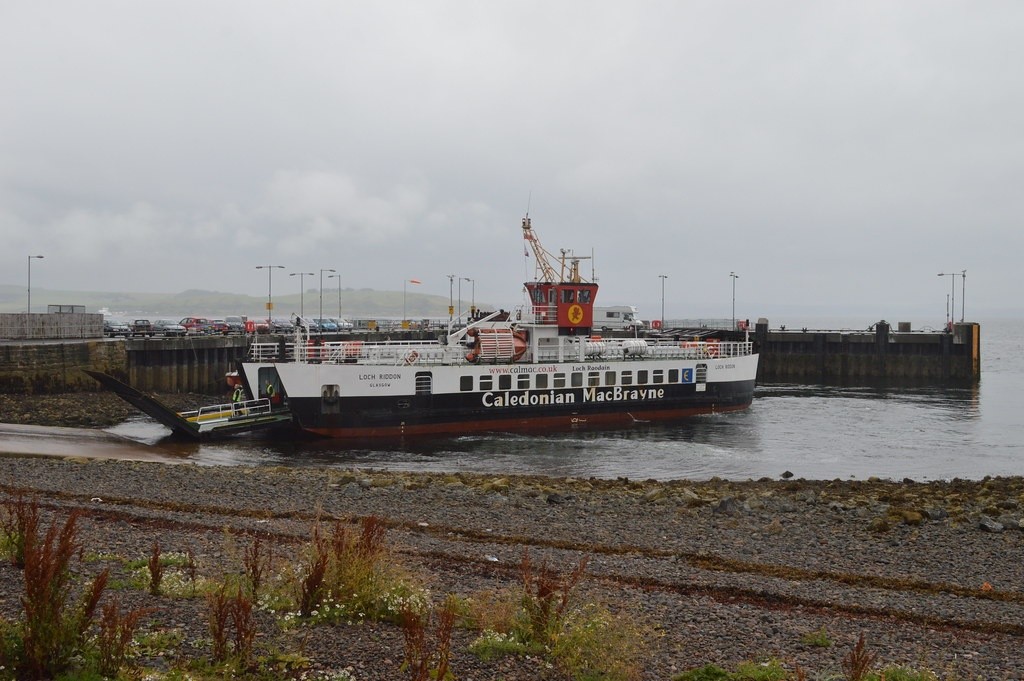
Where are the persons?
[264,380,275,412]
[232,384,243,417]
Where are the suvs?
[128,319,153,337]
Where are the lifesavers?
[404,350,418,363]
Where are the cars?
[331,318,353,331]
[270,318,294,334]
[103,319,131,338]
[313,319,339,332]
[180,316,269,337]
[300,317,317,334]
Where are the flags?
[523,242,530,257]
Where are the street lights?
[329,275,341,319]
[320,269,336,333]
[289,273,315,318]
[446,274,455,322]
[468,279,475,318]
[937,273,966,325]
[255,264,285,334]
[27,256,44,314]
[658,274,669,329]
[458,277,469,330]
[730,271,740,330]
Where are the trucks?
[591,306,643,332]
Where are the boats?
[84,188,759,446]
[463,329,527,363]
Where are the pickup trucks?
[149,320,188,337]
